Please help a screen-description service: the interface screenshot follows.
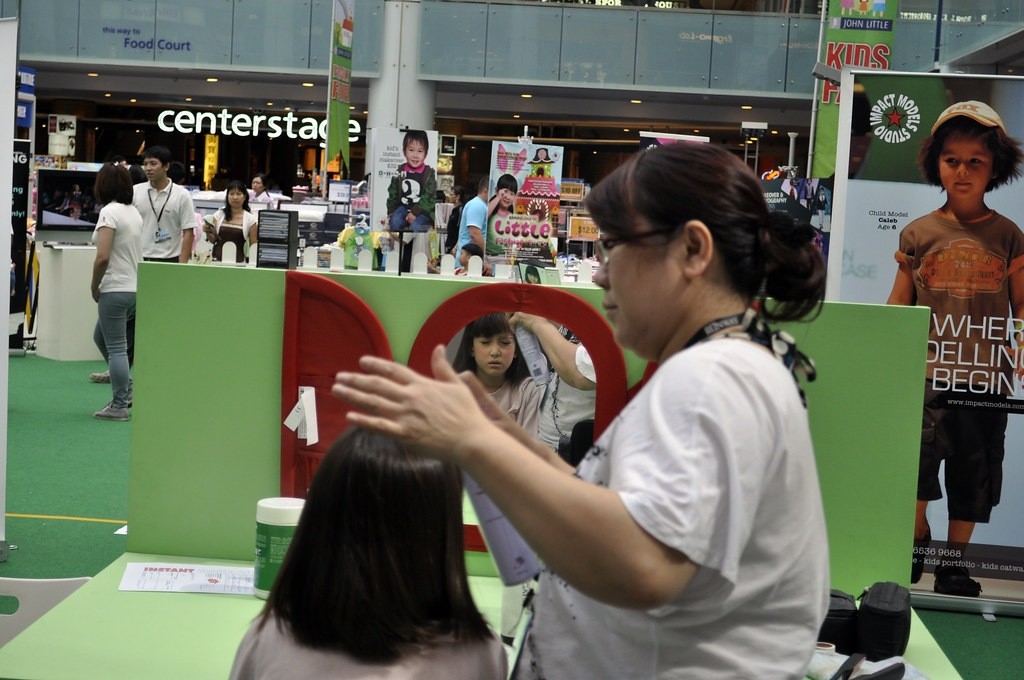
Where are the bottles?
[458,467,542,587]
[515,322,553,388]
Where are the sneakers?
[911,522,931,584]
[93,406,129,420]
[933,566,981,597]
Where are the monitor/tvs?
[36,169,111,232]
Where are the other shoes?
[90,370,111,382]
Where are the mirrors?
[407,282,626,558]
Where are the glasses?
[593,225,678,265]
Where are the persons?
[451,309,539,439]
[90,147,277,420]
[386,129,435,231]
[224,430,510,680]
[443,174,490,277]
[882,97,1024,596]
[506,311,597,455]
[332,143,833,680]
[487,173,517,219]
[525,266,541,284]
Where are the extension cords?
[25,349,37,355]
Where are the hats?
[931,100,1006,133]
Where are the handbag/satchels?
[816,588,857,659]
[856,582,912,663]
